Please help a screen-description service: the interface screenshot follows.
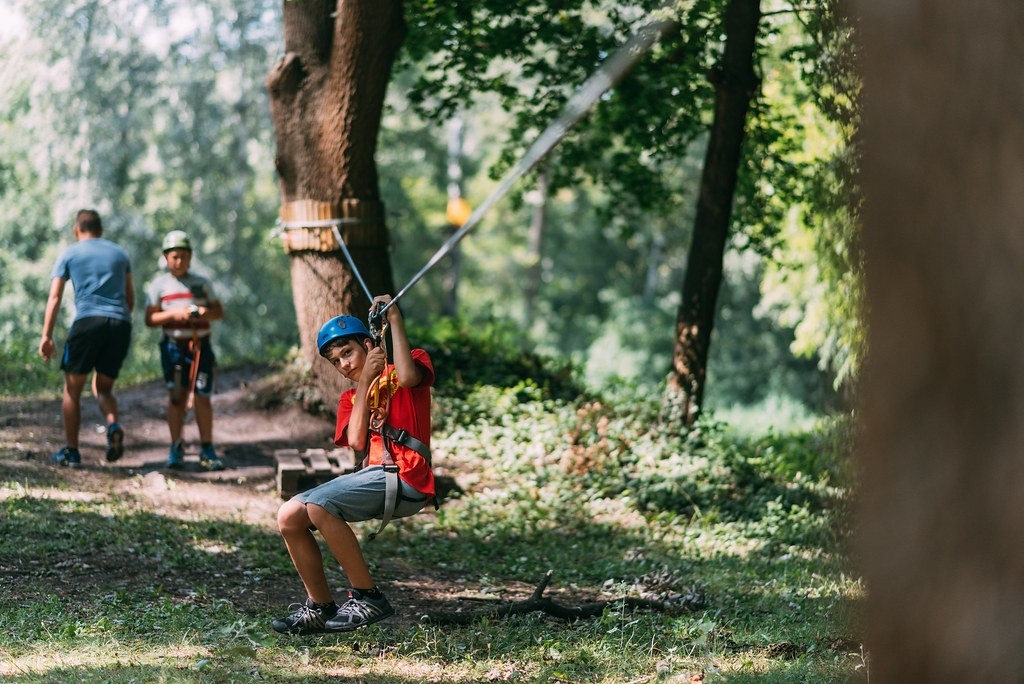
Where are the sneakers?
[271,600,339,635]
[52,446,80,468]
[198,448,223,471]
[106,423,125,462]
[168,439,186,468]
[325,589,395,631]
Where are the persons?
[39,209,134,467]
[272,294,433,634]
[145,230,223,471]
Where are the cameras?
[188,304,199,317]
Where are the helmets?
[161,230,191,255]
[316,316,368,355]
